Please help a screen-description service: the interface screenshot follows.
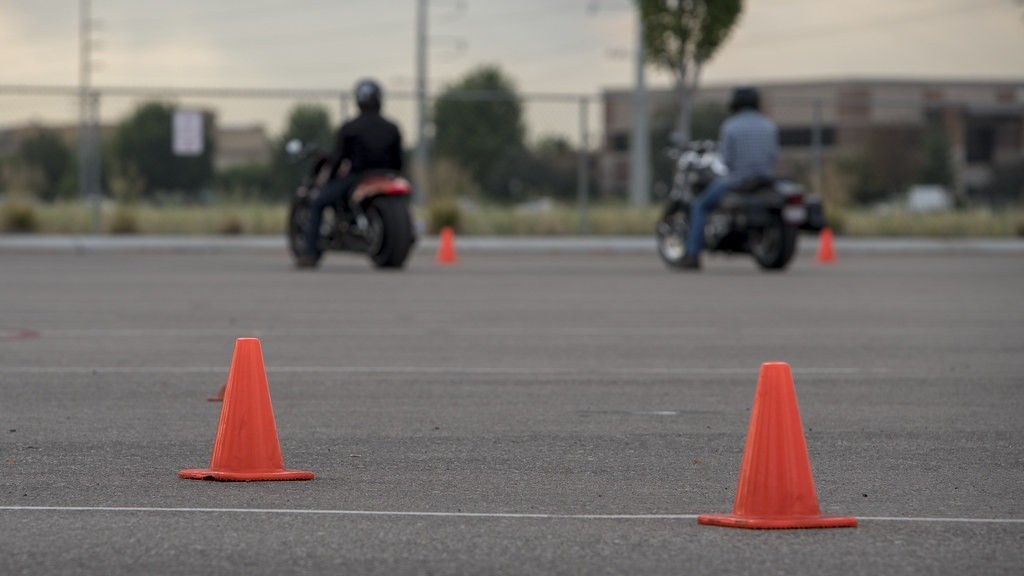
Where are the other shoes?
[674,252,699,269]
[295,253,317,267]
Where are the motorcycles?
[285,137,415,266]
[651,127,807,271]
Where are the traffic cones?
[815,226,837,263]
[694,360,860,530]
[177,336,316,484]
[440,227,456,262]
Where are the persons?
[674,83,781,269]
[298,78,405,270]
